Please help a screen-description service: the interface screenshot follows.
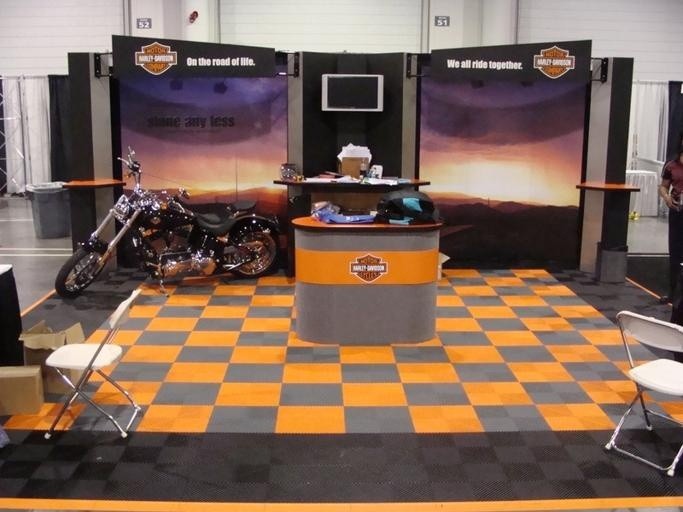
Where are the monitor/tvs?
[322,73,383,113]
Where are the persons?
[657,150,682,304]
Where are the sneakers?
[658,293,673,306]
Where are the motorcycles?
[54,145,281,299]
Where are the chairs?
[42,283,145,440]
[602,310,683,477]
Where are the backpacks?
[374,189,436,225]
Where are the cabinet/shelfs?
[272,169,444,347]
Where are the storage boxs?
[0,319,86,416]
